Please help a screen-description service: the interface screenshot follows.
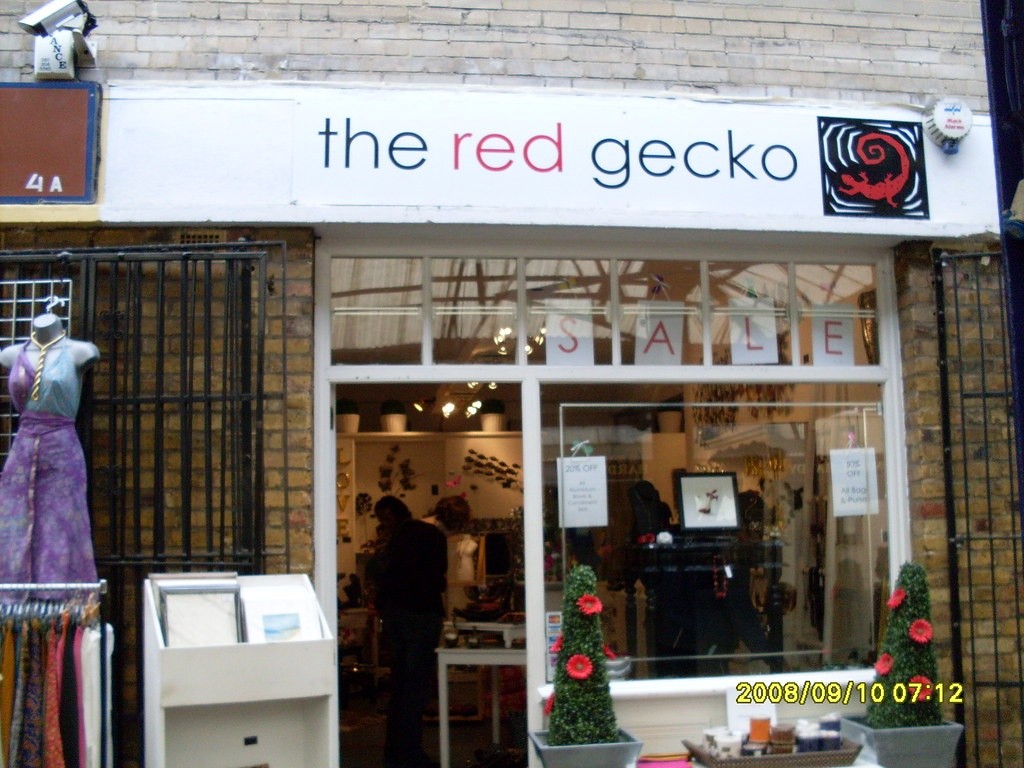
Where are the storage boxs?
[682,736,862,767]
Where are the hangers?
[0,590,101,623]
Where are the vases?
[529,727,643,768]
[842,715,964,768]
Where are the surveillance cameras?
[17,0,97,38]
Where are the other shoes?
[449,704,478,717]
[423,699,438,715]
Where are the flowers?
[865,560,945,727]
[544,564,620,746]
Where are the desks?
[436,647,527,768]
[441,621,526,745]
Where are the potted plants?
[379,400,408,431]
[479,400,507,431]
[337,398,361,431]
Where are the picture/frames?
[677,472,744,532]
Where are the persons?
[364,496,471,767]
[568,527,611,571]
[628,481,673,546]
[0,313,99,603]
